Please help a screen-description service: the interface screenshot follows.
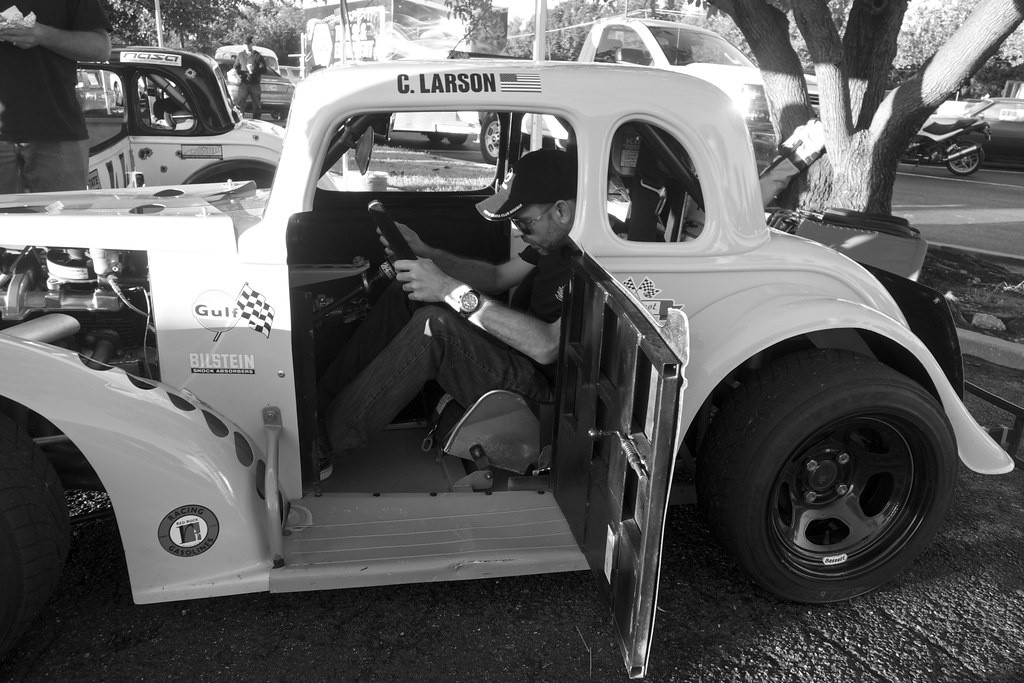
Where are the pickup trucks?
[449,17,822,167]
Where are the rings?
[14,42,15,46]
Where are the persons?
[318,150,577,481]
[234,35,266,119]
[0,0,112,193]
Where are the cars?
[0,58,1019,680]
[271,66,300,84]
[109,71,177,106]
[87,47,287,195]
[214,45,296,120]
[75,68,110,109]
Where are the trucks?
[298,5,483,145]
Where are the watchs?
[458,288,480,319]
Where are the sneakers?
[320,460,333,480]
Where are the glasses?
[511,204,553,234]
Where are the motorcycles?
[899,97,995,175]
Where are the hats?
[475,148,577,221]
